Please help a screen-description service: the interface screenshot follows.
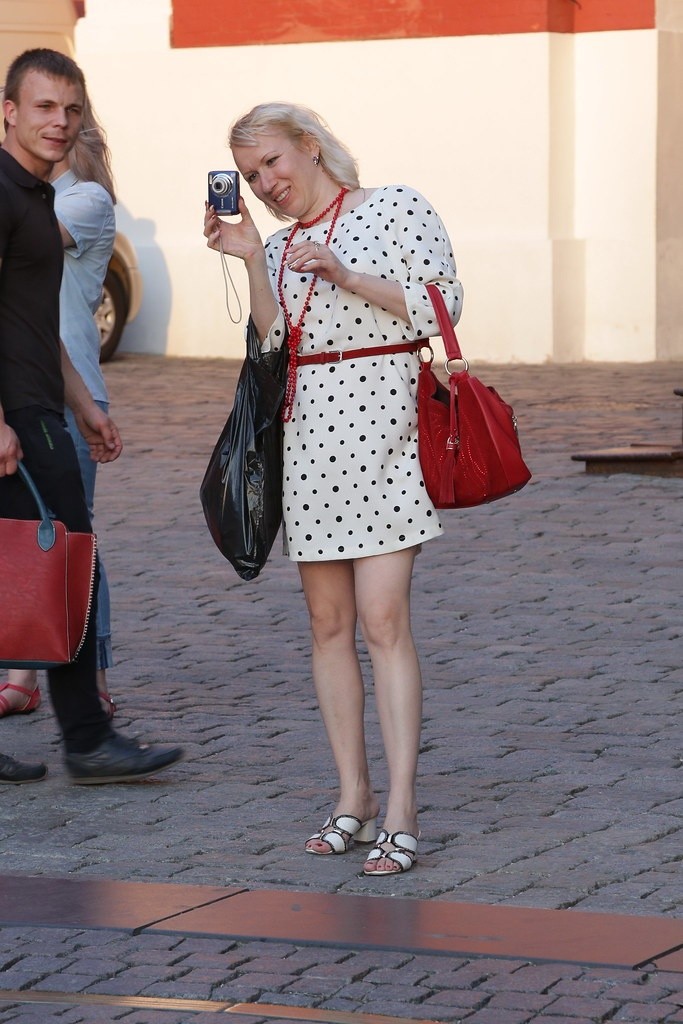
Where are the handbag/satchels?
[0,461,97,669]
[199,312,291,581]
[417,285,532,509]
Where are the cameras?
[208,170,241,216]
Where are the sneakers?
[63,727,185,785]
[0,752,48,785]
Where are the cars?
[92,229,145,363]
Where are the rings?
[313,241,320,247]
[218,221,222,224]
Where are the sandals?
[0,681,41,719]
[99,692,116,721]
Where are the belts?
[298,342,419,366]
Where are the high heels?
[305,812,377,854]
[364,829,421,875]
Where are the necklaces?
[277,187,349,423]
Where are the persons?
[0,48,184,786]
[203,99,463,875]
[0,86,118,720]
